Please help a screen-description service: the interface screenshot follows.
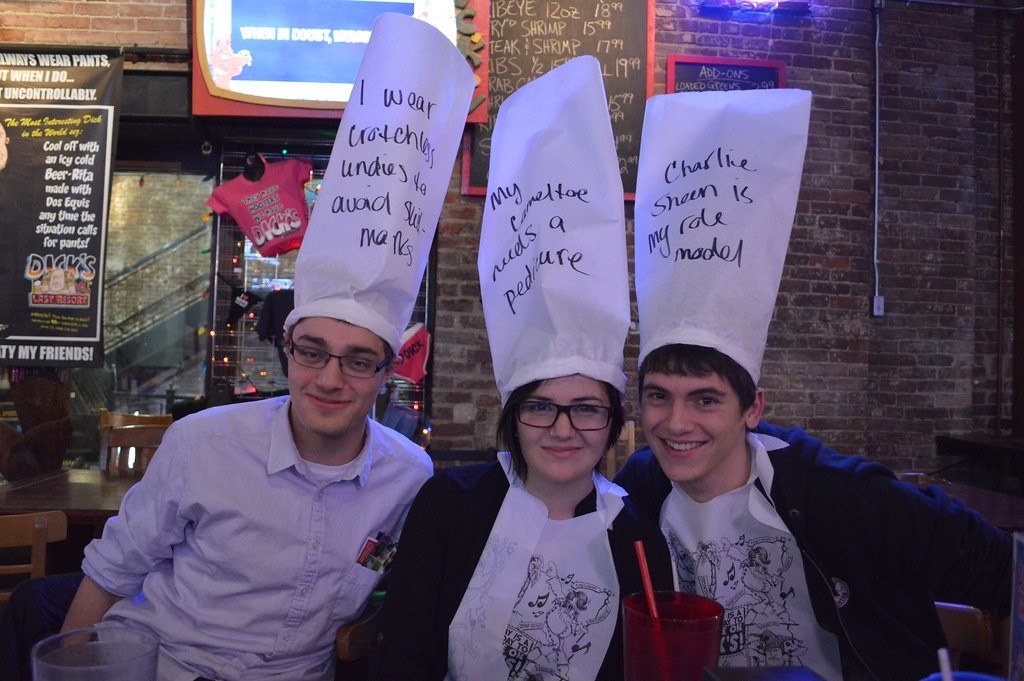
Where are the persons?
[362,375,674,681]
[610,343,1024,681]
[58,314,435,681]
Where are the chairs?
[0,408,176,612]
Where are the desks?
[0,464,146,541]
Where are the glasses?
[290,337,391,379]
[518,401,615,431]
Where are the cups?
[621,590,724,681]
[32,625,161,681]
[920,671,1010,681]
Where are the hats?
[479,55,628,410]
[636,89,812,385]
[283,13,475,356]
